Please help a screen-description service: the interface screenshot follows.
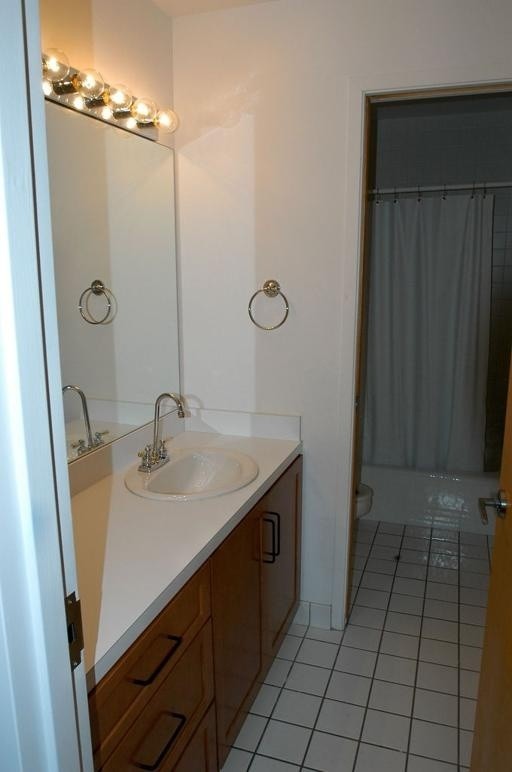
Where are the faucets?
[63,384,93,449]
[152,394,185,447]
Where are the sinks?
[355,483,374,521]
[125,450,258,503]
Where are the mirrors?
[46,101,187,464]
